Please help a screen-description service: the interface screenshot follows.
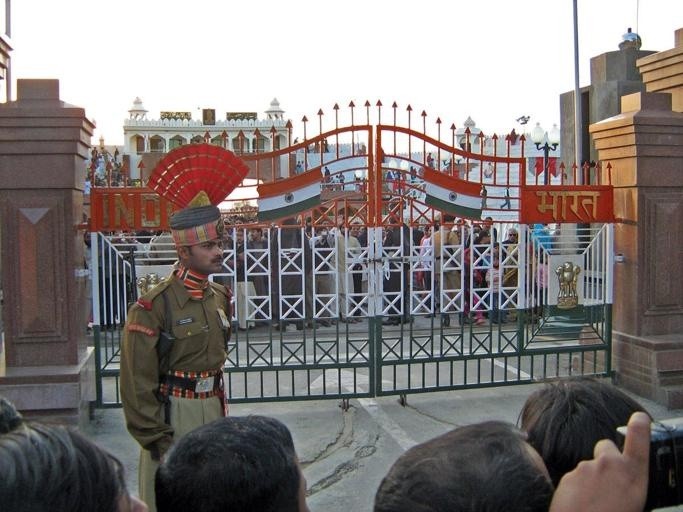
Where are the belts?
[157,370,225,394]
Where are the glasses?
[509,234,516,236]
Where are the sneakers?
[458,315,509,325]
[423,313,435,318]
[233,312,414,332]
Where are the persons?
[517,377,654,490]
[82,138,547,333]
[373,421,555,512]
[549,411,650,512]
[120,206,233,512]
[154,415,310,512]
[0,420,148,512]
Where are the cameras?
[613,415,683,509]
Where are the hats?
[168,205,225,248]
